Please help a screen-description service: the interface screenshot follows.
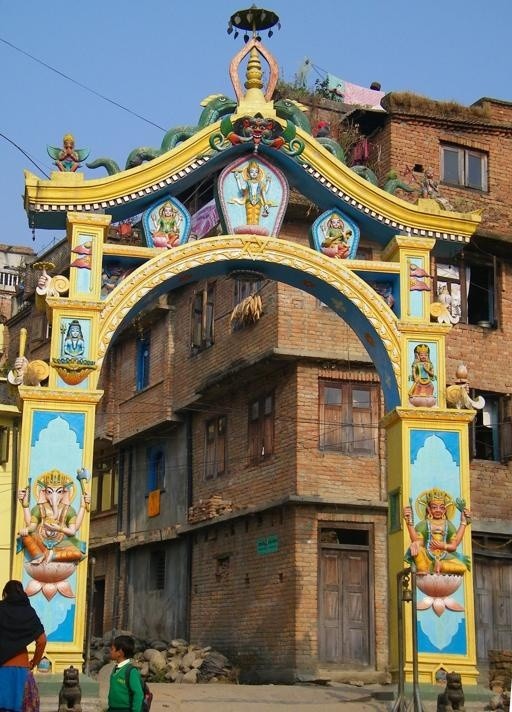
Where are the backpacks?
[124,662,154,712]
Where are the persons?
[409,344,435,395]
[402,487,472,576]
[103,633,147,711]
[227,160,277,227]
[418,165,438,197]
[56,134,79,172]
[64,319,85,359]
[150,203,183,248]
[1,578,47,712]
[320,213,353,260]
